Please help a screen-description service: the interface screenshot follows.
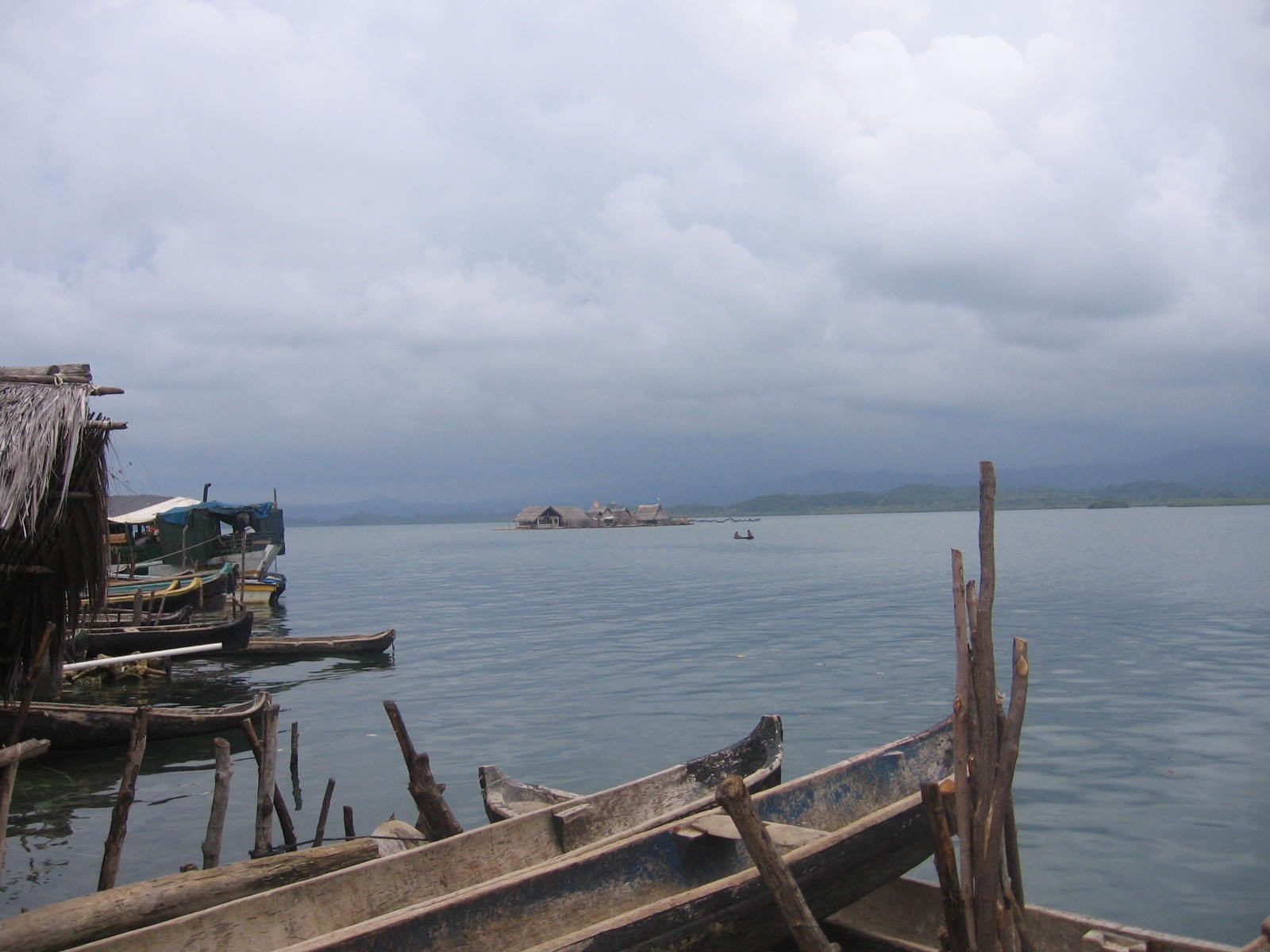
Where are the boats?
[470,751,1243,950]
[60,711,787,952]
[280,690,1012,952]
[734,535,754,539]
[64,482,398,659]
[0,689,275,747]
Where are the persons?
[734,529,754,538]
